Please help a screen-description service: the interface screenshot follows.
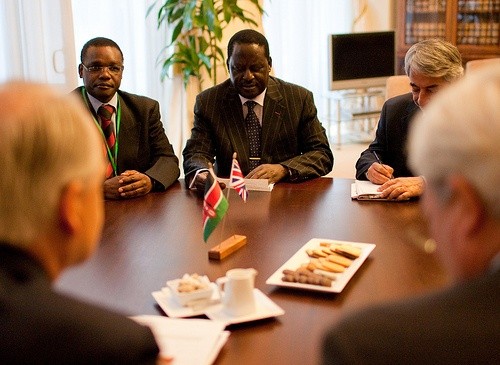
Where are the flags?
[229,157,249,204]
[202,168,229,243]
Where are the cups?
[215,267,258,315]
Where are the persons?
[70,37,180,201]
[0,80,161,365]
[356,38,465,200]
[182,28,334,193]
[317,62,500,365]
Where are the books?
[350,179,410,200]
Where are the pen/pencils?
[372,150,393,180]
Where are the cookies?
[280,242,360,290]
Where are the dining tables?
[52,179,456,365]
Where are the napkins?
[127,315,231,365]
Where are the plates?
[204,288,285,326]
[152,281,224,319]
[265,237,378,294]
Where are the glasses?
[82,62,124,73]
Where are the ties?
[96,104,117,180]
[243,101,263,173]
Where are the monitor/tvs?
[328,30,396,94]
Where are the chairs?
[373,75,415,140]
[465,57,500,77]
